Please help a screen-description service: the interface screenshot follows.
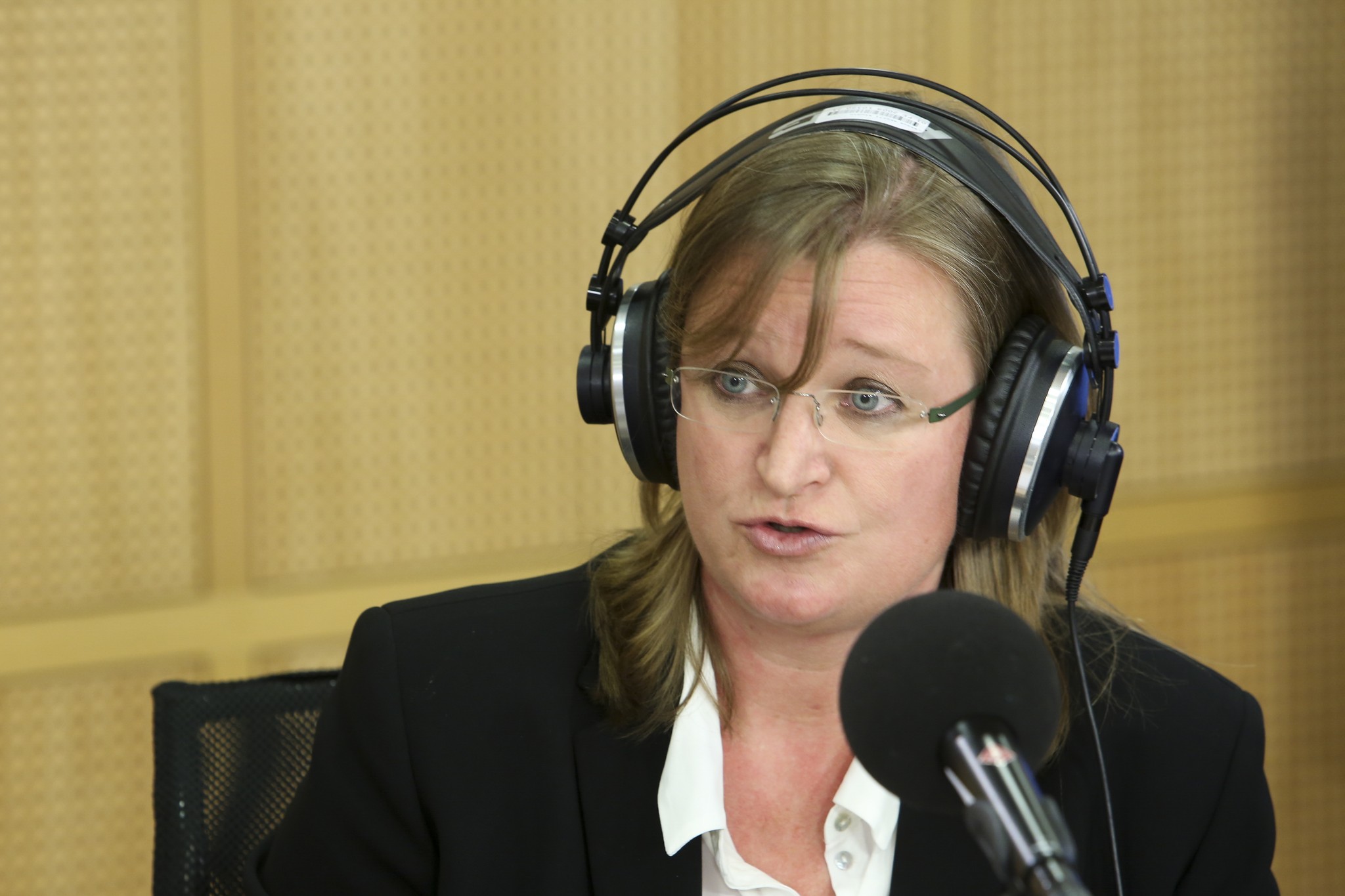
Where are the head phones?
[575,67,1126,605]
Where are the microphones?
[839,589,1092,896]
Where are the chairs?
[150,674,338,896]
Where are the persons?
[242,69,1280,896]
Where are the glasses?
[662,366,986,448]
